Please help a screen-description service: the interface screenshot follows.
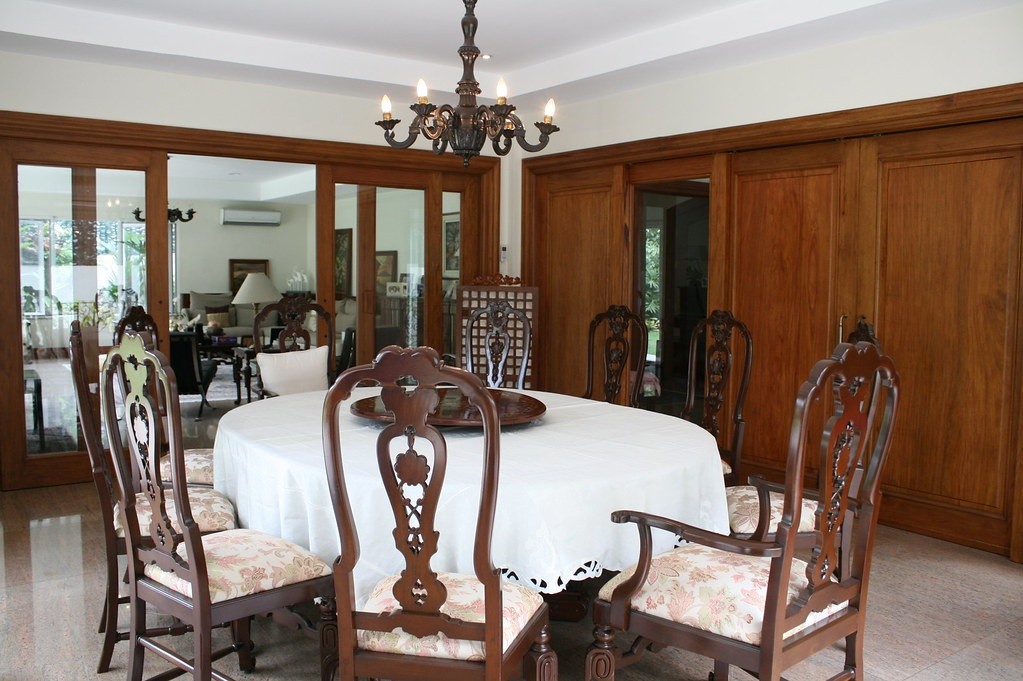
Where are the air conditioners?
[220,207,282,228]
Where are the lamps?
[230,271,282,350]
[374,0,562,168]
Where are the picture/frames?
[386,282,401,296]
[375,251,398,295]
[401,283,409,297]
[442,212,460,280]
[228,258,269,291]
[334,227,353,298]
[399,272,414,285]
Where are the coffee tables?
[198,343,247,365]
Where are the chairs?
[322,343,559,681]
[100,330,338,681]
[581,305,649,410]
[69,319,255,674]
[169,331,219,422]
[678,310,754,488]
[252,290,336,401]
[112,304,214,586]
[583,339,901,681]
[723,312,877,577]
[465,300,532,390]
[329,325,404,386]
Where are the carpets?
[26,425,77,453]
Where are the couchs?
[271,299,358,358]
[180,307,279,338]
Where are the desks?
[212,384,730,681]
[24,369,47,452]
[230,345,290,405]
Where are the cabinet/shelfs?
[380,294,452,362]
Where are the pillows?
[335,311,356,335]
[237,307,279,327]
[189,291,233,316]
[190,308,236,327]
[307,310,317,332]
[204,305,231,327]
[256,345,329,398]
